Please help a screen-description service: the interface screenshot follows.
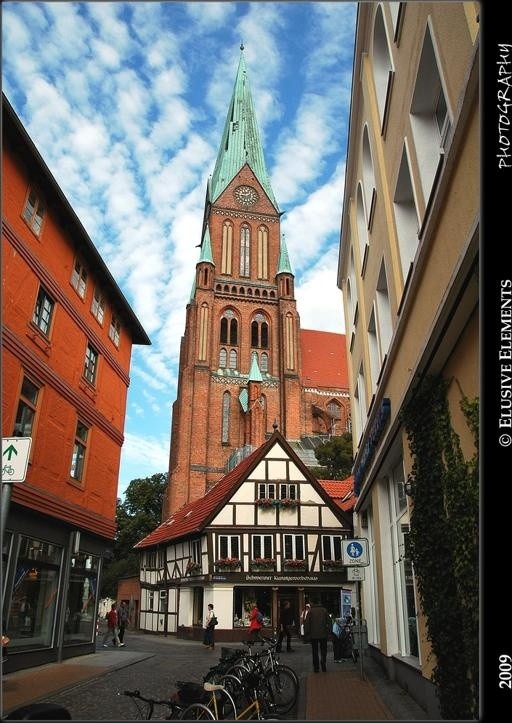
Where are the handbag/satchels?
[300,624,304,636]
[257,612,263,623]
[209,617,218,626]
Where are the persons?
[304,595,332,674]
[112,601,130,647]
[274,600,295,653]
[332,615,353,663]
[201,604,216,650]
[246,602,267,646]
[101,603,126,649]
[299,603,313,644]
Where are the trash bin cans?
[332,617,356,663]
[7,702,72,720]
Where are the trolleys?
[349,617,371,662]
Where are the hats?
[304,604,310,608]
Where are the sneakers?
[200,643,214,650]
[101,638,126,648]
[287,648,295,652]
[276,647,285,653]
[320,660,326,672]
[314,669,319,673]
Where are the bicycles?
[124,636,300,720]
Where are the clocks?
[233,185,259,207]
